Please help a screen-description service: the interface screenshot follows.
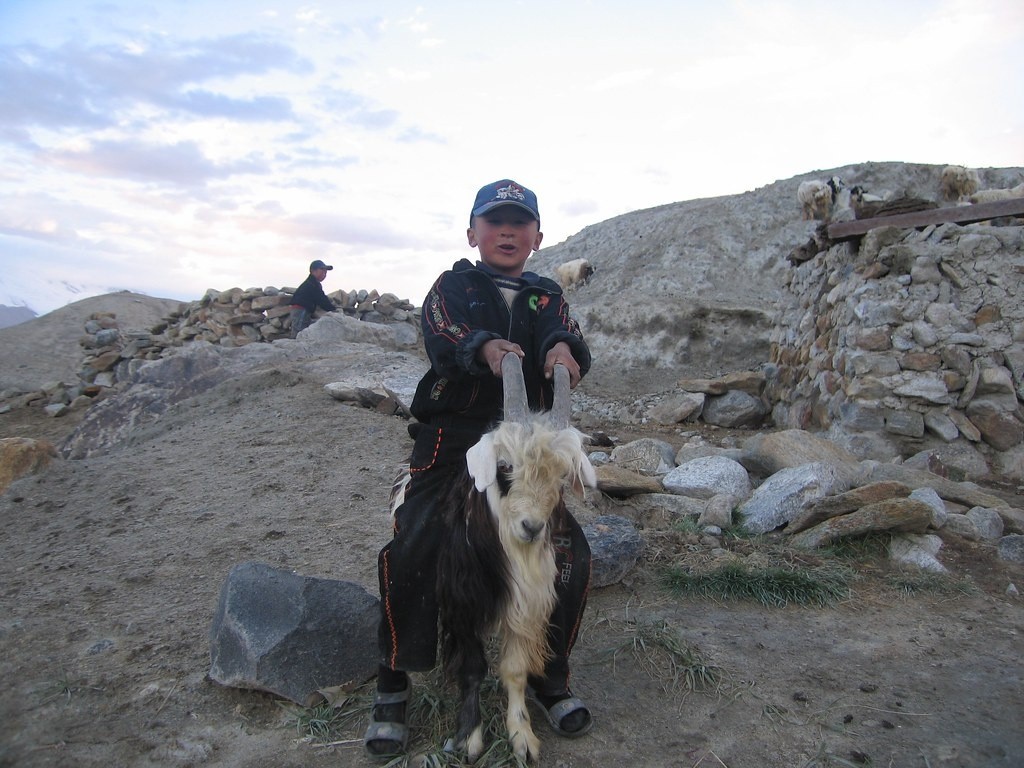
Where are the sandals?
[362,674,413,761]
[526,682,593,738]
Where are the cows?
[796,174,884,222]
[554,257,597,294]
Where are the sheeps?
[427,349,599,764]
[942,165,1024,205]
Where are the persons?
[289,260,335,339]
[365,178,592,759]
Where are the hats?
[469,179,540,232]
[310,260,333,270]
